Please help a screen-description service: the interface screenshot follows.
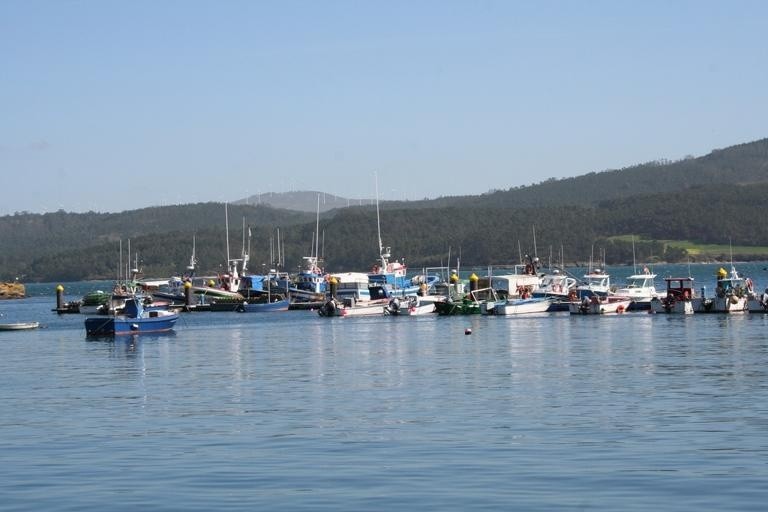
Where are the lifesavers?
[313,267,321,274]
[747,278,753,290]
[387,263,392,273]
[553,284,563,294]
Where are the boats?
[0,321,39,330]
